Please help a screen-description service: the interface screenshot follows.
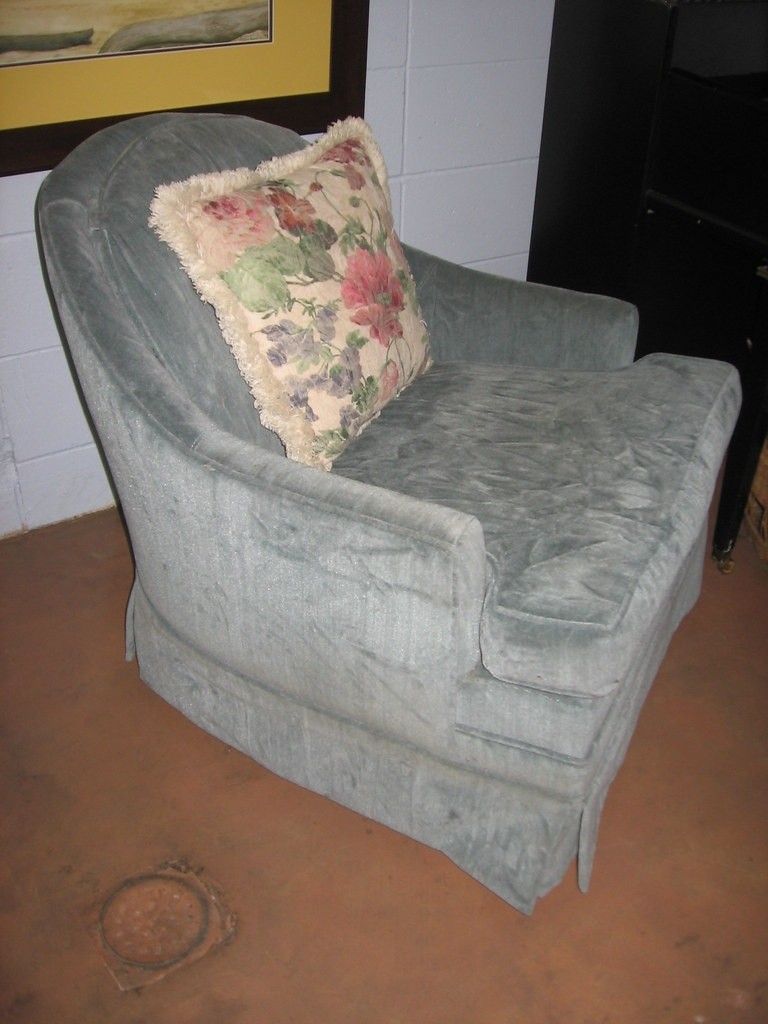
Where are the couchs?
[31,107,747,917]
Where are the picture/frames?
[0,0,373,179]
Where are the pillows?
[149,117,439,470]
